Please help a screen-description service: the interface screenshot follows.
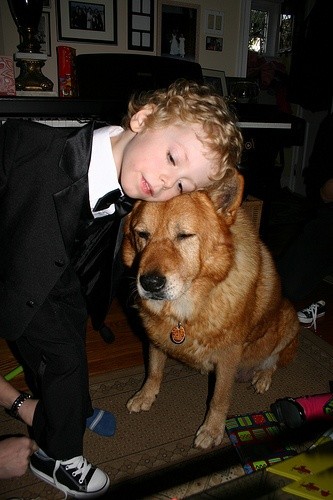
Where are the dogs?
[122,174,300,450]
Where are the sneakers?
[28,448,110,497]
[297,299,326,323]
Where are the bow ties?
[93,188,135,218]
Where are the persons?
[0,375,38,479]
[169,29,182,56]
[178,34,186,59]
[0,81,241,496]
[69,4,104,31]
[207,37,222,51]
[294,176,333,325]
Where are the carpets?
[0,326,333,500]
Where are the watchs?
[10,392,32,419]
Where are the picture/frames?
[156,0,201,63]
[55,0,117,46]
